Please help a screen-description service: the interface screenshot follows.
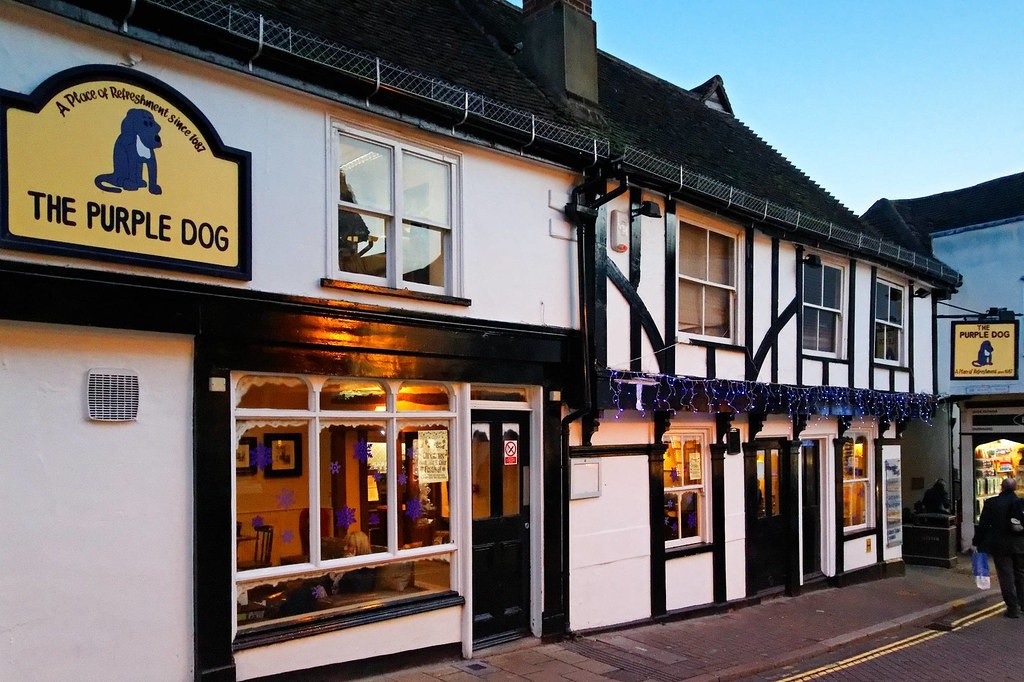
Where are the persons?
[973,479,1024,618]
[757,479,764,515]
[345,523,372,556]
[339,174,369,276]
[922,478,950,514]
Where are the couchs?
[264,536,427,621]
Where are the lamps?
[635,201,663,219]
[801,254,822,269]
[914,287,932,299]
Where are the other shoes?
[1002,611,1019,618]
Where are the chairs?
[238,525,274,572]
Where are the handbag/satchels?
[1008,498,1024,535]
[971,548,990,590]
